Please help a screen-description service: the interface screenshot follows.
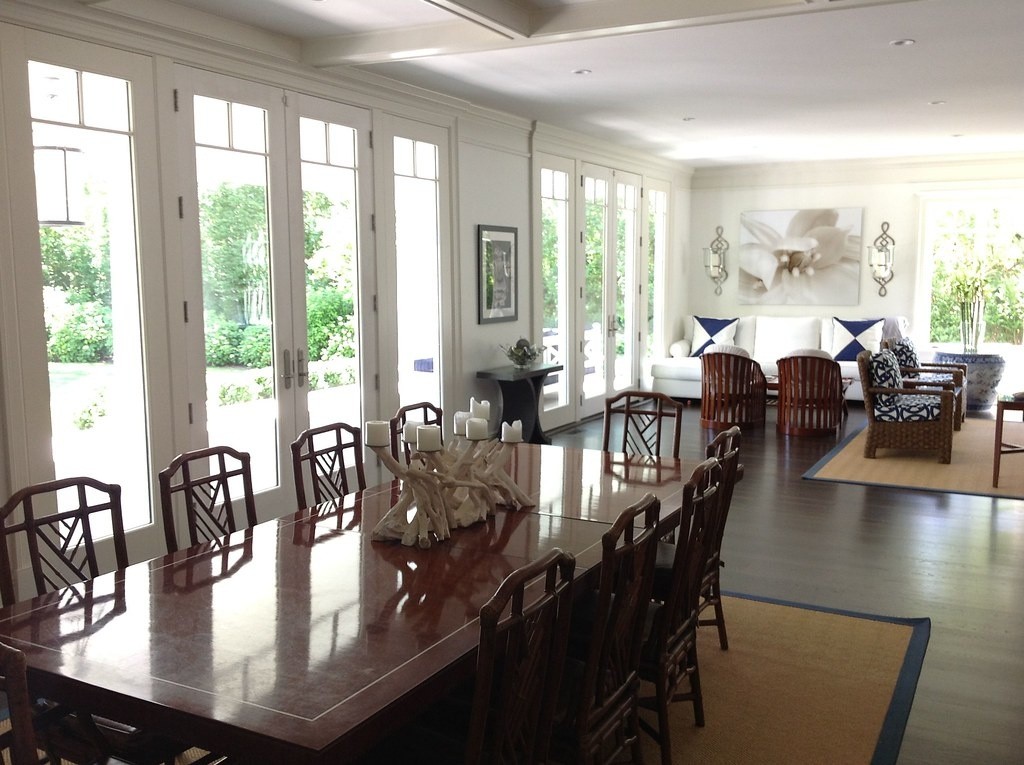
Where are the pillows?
[685,315,739,356]
[897,336,921,376]
[831,317,883,361]
[873,348,902,407]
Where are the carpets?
[0,571,930,765]
[803,414,1024,501]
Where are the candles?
[466,418,489,438]
[469,396,491,420]
[502,419,522,442]
[454,411,474,434]
[415,424,441,449]
[367,421,390,446]
[402,419,424,442]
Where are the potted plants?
[918,233,1024,411]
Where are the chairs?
[851,352,955,464]
[390,345,768,764]
[773,348,848,437]
[290,423,366,507]
[0,477,227,765]
[157,445,258,553]
[0,643,113,765]
[881,337,968,431]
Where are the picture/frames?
[477,224,523,323]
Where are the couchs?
[652,311,911,406]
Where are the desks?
[989,396,1024,488]
[0,447,744,765]
[474,363,565,445]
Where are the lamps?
[703,225,731,295]
[866,221,900,295]
